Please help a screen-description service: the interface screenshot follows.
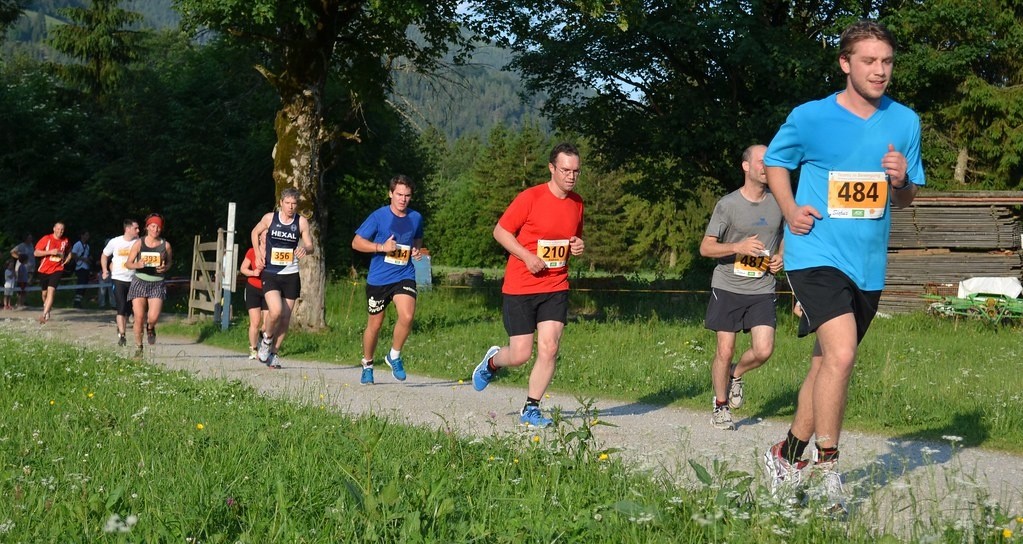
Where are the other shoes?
[4,305,52,324]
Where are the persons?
[470,141,584,429]
[251,187,314,369]
[351,174,424,386]
[127,212,173,360]
[72,230,92,309]
[10,233,39,311]
[3,260,17,311]
[763,18,925,523]
[101,217,140,347]
[95,239,117,310]
[699,144,786,431]
[240,228,272,360]
[34,222,72,325]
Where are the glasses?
[552,163,581,176]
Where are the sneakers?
[258,328,274,363]
[520,406,552,428]
[811,460,848,521]
[361,359,374,384]
[763,440,809,507]
[117,328,127,347]
[727,363,746,409]
[131,343,144,360]
[249,345,258,359]
[472,346,501,392]
[385,348,406,381]
[268,350,281,369]
[711,395,735,431]
[146,322,156,345]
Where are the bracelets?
[375,242,378,253]
[381,244,383,253]
